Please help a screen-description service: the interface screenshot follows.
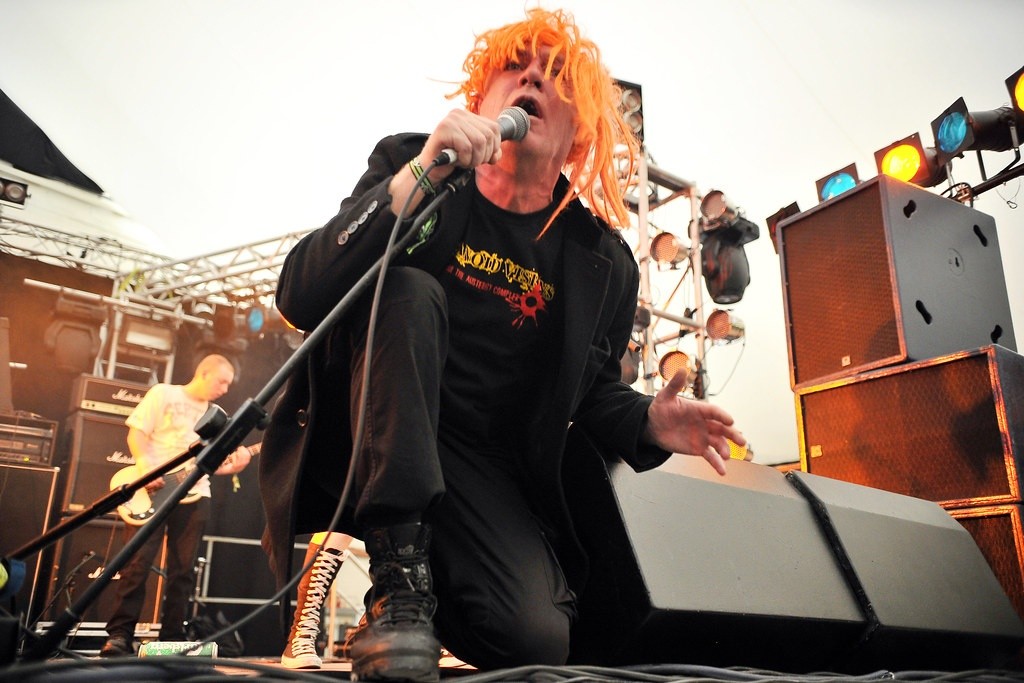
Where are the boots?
[350,520,438,680]
[280,541,349,669]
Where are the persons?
[258,10,748,683]
[97,354,251,657]
[280,530,353,673]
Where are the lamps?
[647,65,1024,395]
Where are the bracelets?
[406,157,439,255]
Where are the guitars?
[108,438,262,526]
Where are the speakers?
[538,422,868,668]
[795,343,1024,509]
[945,503,1024,626]
[786,469,1024,675]
[776,174,1018,391]
[50,410,135,519]
[26,516,168,638]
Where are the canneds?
[138,642,218,668]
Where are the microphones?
[433,105,530,166]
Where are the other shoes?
[99,635,135,655]
